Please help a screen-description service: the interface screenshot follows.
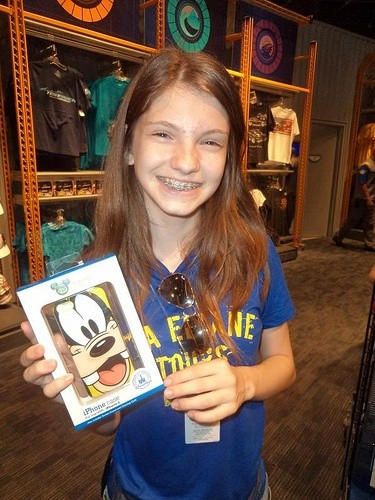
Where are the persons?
[332,144,375,252]
[19,48,297,500]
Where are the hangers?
[46,46,65,70]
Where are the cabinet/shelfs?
[0,0,317,307]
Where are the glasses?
[157,272,211,358]
